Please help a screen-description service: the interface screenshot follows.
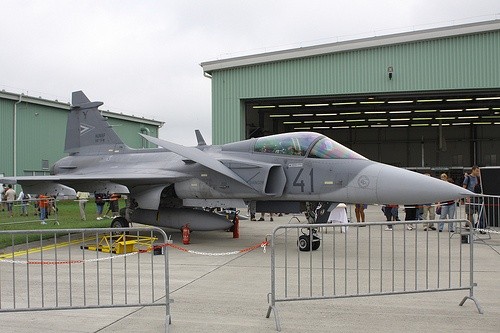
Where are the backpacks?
[23,194,30,202]
[4,188,9,192]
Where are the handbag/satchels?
[466,213,478,224]
[436,206,441,214]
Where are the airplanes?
[0,91,500,252]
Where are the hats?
[3,184,6,187]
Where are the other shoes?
[41,222,47,225]
[439,230,442,232]
[270,217,273,221]
[423,227,427,231]
[258,218,264,221]
[26,213,28,217]
[429,226,436,230]
[450,230,454,233]
[96,215,120,221]
[385,225,392,231]
[407,227,412,231]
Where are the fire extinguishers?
[233,210,241,238]
[181,224,192,244]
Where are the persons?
[39,193,49,225]
[355,204,369,227]
[404,204,416,230]
[0,184,5,211]
[463,171,471,179]
[415,204,423,220]
[440,173,447,180]
[463,165,481,229]
[95,191,107,220]
[17,191,32,216]
[439,177,456,232]
[257,212,274,221]
[4,185,16,217]
[423,172,437,231]
[75,192,91,222]
[382,205,394,230]
[34,193,39,207]
[110,193,122,219]
[390,204,400,221]
